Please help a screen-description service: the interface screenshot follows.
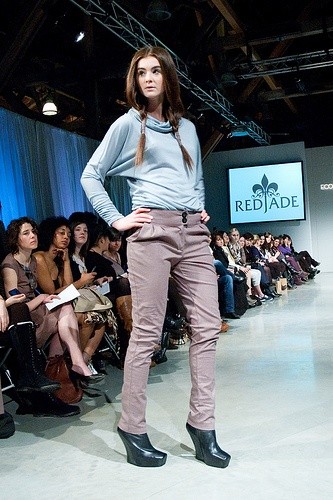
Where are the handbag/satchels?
[260,277,288,299]
[44,354,83,405]
[71,286,113,313]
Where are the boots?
[7,321,61,392]
[34,392,80,417]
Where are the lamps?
[21,82,58,116]
[145,0,172,21]
[219,121,232,139]
[55,13,86,44]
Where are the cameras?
[9,288,22,296]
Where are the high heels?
[117,427,167,467]
[186,422,231,468]
[71,369,105,390]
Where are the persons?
[0,211,321,440]
[80,47,231,467]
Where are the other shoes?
[220,322,229,333]
[225,292,273,319]
[151,313,193,364]
[0,411,16,439]
[287,261,321,290]
